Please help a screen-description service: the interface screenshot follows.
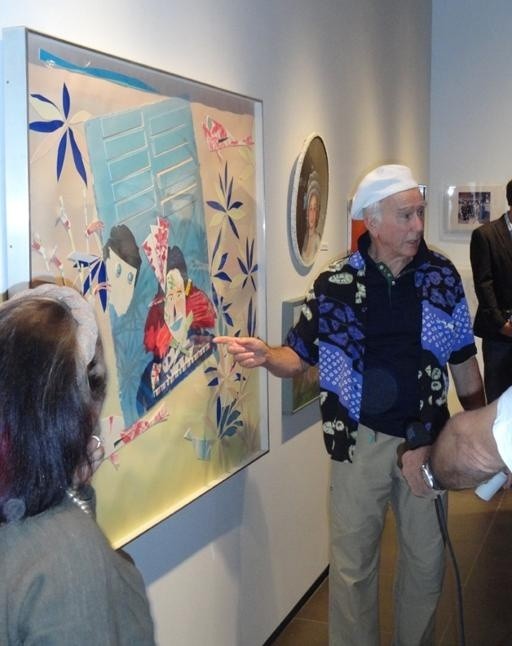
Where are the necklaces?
[41,469,101,523]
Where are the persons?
[103,222,141,317]
[400,385,512,502]
[215,165,511,645]
[471,181,511,401]
[0,278,154,646]
[137,244,216,418]
[301,170,322,264]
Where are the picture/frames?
[291,132,329,267]
[281,295,320,416]
[4,25,269,551]
[439,182,506,242]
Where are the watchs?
[418,455,442,495]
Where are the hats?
[348,161,421,221]
